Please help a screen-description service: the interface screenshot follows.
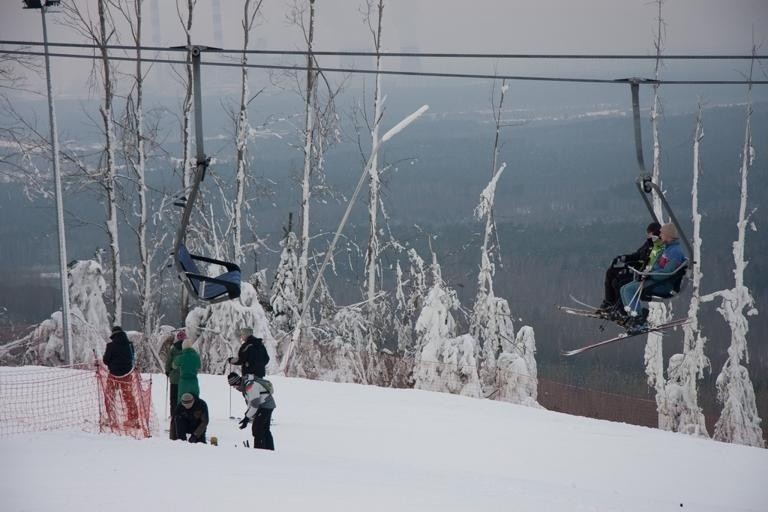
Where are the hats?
[178,331,186,339]
[661,224,679,239]
[181,393,194,405]
[241,328,253,336]
[228,372,241,386]
[182,338,192,349]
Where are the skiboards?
[554,293,695,358]
[230,415,280,425]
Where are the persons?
[227,326,270,381]
[165,331,190,440]
[171,338,201,402]
[169,391,209,444]
[620,223,686,334]
[103,325,139,428]
[596,221,662,318]
[227,372,275,451]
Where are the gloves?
[238,417,249,429]
